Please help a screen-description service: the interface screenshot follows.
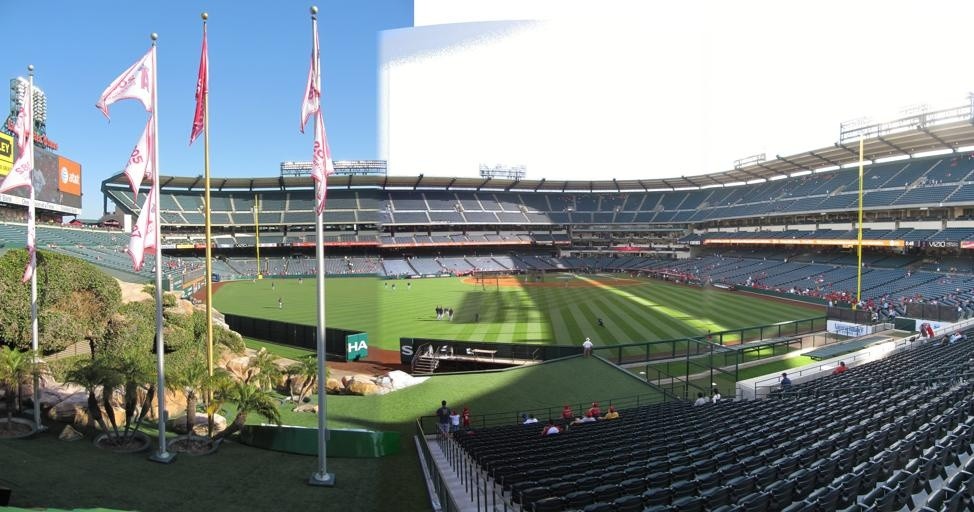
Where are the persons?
[948,331,962,342]
[147,253,434,296]
[581,337,593,358]
[710,387,721,406]
[434,243,974,322]
[780,372,791,389]
[933,334,948,347]
[925,322,934,338]
[435,399,623,439]
[832,361,848,375]
[596,318,605,328]
[917,324,928,345]
[278,297,282,309]
[692,392,706,407]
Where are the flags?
[310,108,336,213]
[96,50,155,123]
[121,113,159,207]
[299,22,322,134]
[125,176,161,272]
[0,76,34,197]
[189,20,208,147]
